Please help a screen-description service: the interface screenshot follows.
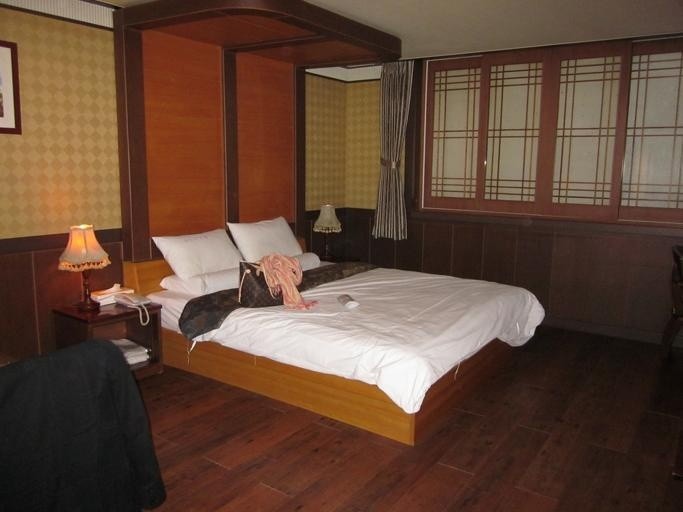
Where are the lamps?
[0,339,172,512]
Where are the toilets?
[114,292,151,307]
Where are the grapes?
[56,302,167,381]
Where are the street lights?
[237,260,284,308]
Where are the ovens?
[120,239,545,450]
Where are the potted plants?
[1,39,23,137]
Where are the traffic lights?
[153,214,306,277]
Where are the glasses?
[313,205,340,260]
[55,225,111,308]
